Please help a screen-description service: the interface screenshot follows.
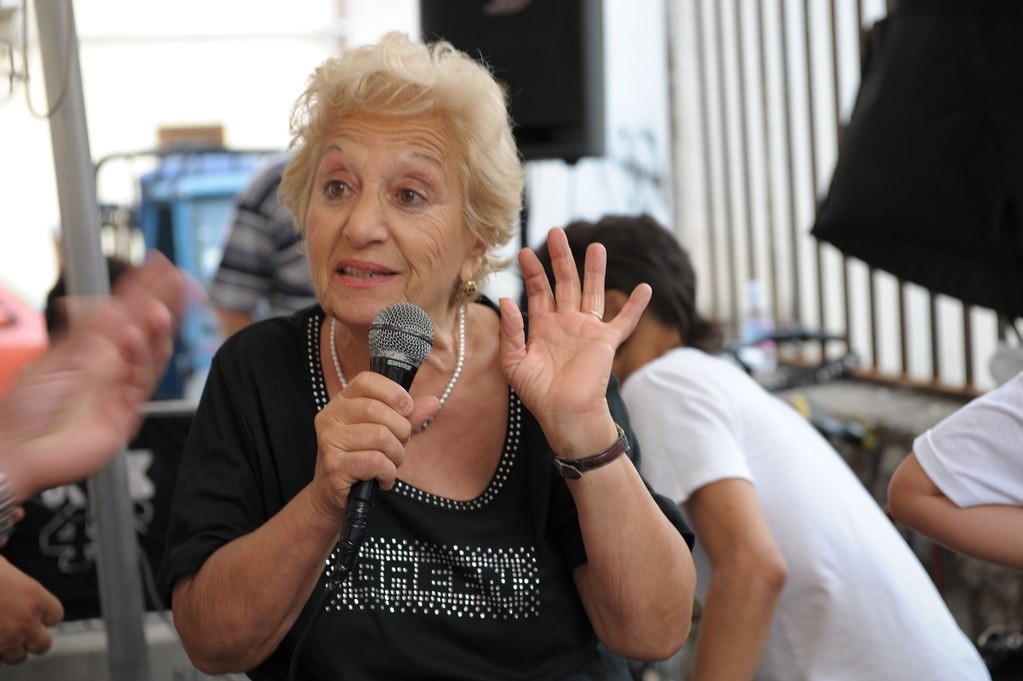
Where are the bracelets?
[552,423,630,480]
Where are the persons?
[522,214,991,681]
[202,160,319,340]
[886,369,1022,570]
[151,33,698,681]
[1,249,201,665]
[43,256,193,401]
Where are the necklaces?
[330,307,465,441]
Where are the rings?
[583,310,602,320]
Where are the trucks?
[89,152,282,402]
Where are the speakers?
[809,0,1023,318]
[420,0,605,166]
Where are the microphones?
[324,300,434,599]
